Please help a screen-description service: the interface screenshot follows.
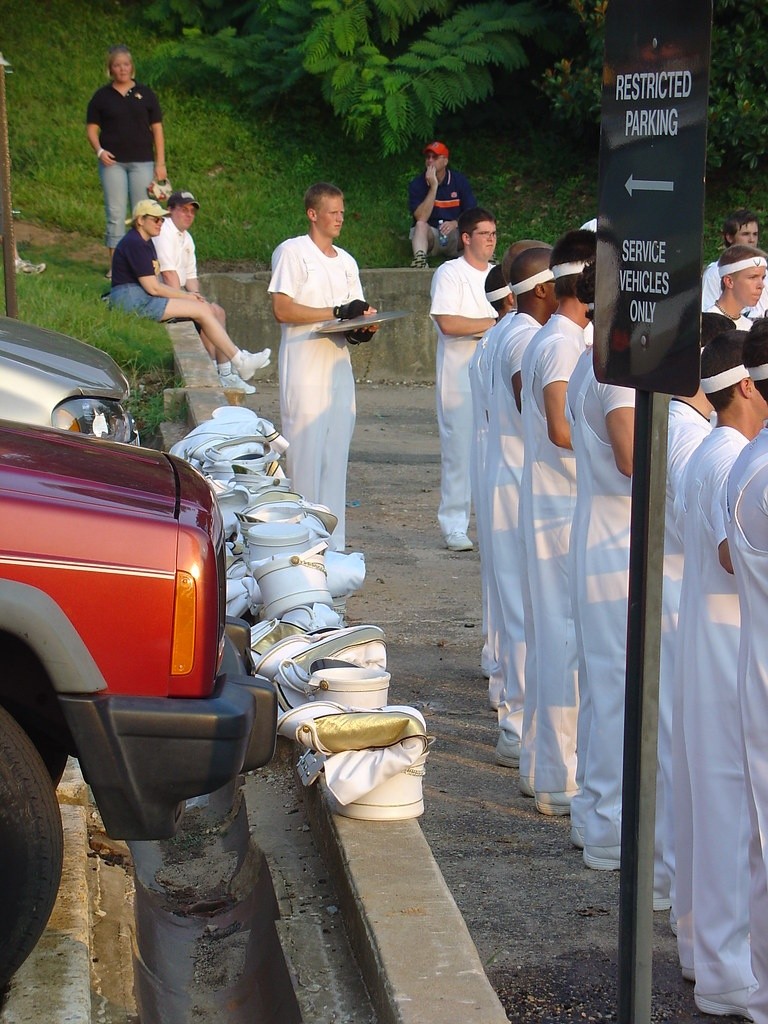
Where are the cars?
[0,314,141,446]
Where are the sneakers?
[533,791,579,816]
[570,827,583,846]
[694,980,759,1021]
[442,532,474,551]
[517,775,536,796]
[230,358,271,374]
[236,348,272,381]
[14,258,45,275]
[219,374,256,394]
[494,730,521,768]
[410,251,429,268]
[583,843,621,872]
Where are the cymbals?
[311,309,416,335]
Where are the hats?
[167,191,200,209]
[125,199,171,225]
[169,406,430,820]
[422,141,448,158]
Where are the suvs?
[0,416,275,987]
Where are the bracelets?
[96,149,105,158]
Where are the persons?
[268,184,380,552]
[0,50,47,277]
[408,142,477,268]
[108,190,271,396]
[85,48,167,281]
[429,208,768,1024]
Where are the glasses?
[425,154,447,160]
[143,216,164,223]
[468,230,498,238]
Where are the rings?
[447,227,450,230]
